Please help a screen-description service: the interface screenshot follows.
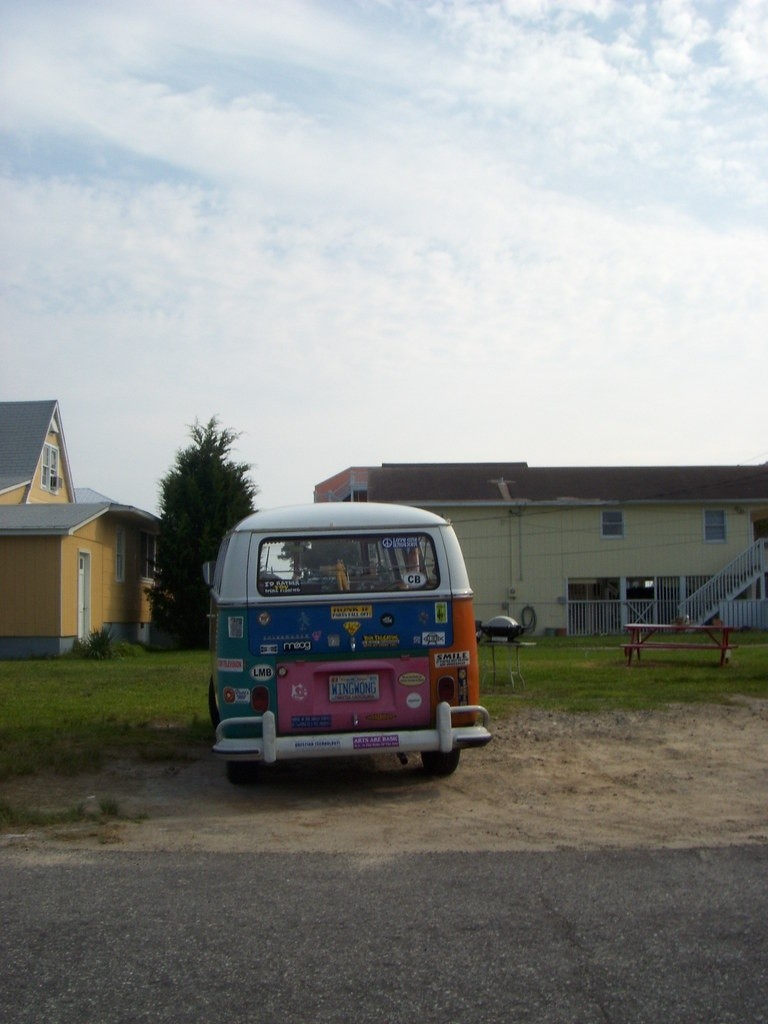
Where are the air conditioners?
[51,475,63,491]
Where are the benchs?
[620,642,738,650]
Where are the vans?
[201,502,491,786]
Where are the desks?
[625,623,733,667]
[479,641,538,691]
